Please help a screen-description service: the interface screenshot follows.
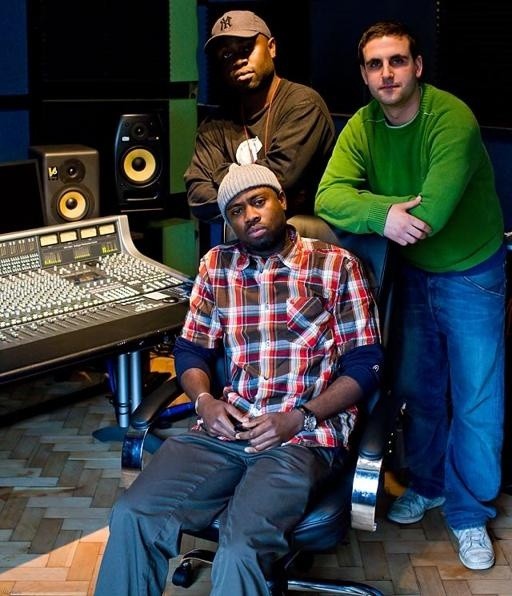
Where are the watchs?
[296,405,318,434]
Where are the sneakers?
[454,526,495,569]
[389,492,445,522]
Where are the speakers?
[111,115,168,216]
[30,142,98,224]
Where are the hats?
[218,165,282,227]
[203,11,272,51]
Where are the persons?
[184,10,334,223]
[93,160,383,596]
[314,24,509,572]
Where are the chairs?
[117,235,393,596]
